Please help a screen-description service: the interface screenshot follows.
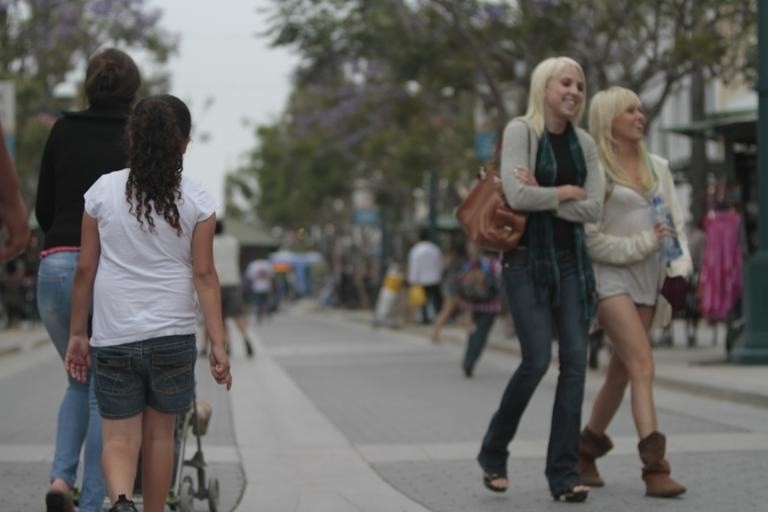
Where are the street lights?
[403,80,462,245]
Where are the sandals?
[549,482,591,502]
[479,453,509,493]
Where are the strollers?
[73,370,223,511]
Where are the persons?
[0,130,31,261]
[575,86,692,498]
[63,94,232,511]
[198,220,276,357]
[406,228,505,378]
[35,49,141,512]
[476,56,607,503]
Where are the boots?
[571,426,615,487]
[637,431,688,498]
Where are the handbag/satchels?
[451,164,529,254]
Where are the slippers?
[44,474,75,512]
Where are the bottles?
[649,195,683,261]
